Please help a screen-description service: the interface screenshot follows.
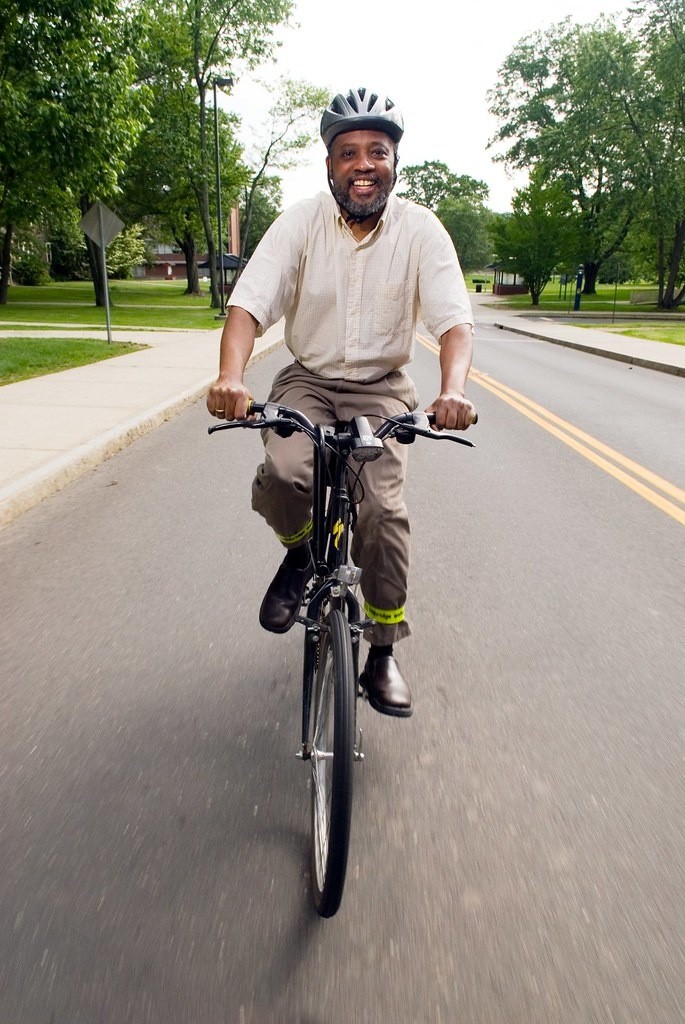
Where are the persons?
[205,86,478,722]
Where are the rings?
[214,409,224,413]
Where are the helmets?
[320,89,403,150]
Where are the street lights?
[212,74,234,317]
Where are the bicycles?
[207,398,483,918]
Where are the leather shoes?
[360,652,413,717]
[259,554,315,634]
